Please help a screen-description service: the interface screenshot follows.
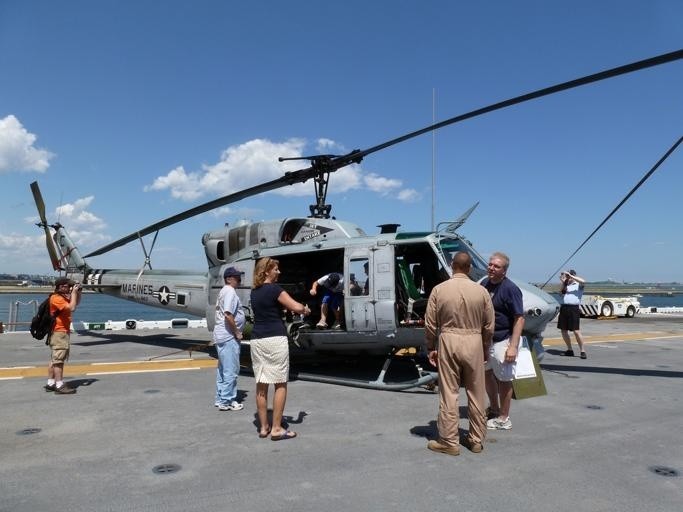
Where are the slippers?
[261,427,271,438]
[271,428,297,440]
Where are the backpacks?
[29,291,67,340]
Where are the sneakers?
[466,435,482,453]
[46,384,56,392]
[214,398,244,411]
[486,416,512,430]
[428,439,460,456]
[54,385,76,395]
[482,407,501,420]
[560,350,574,356]
[332,320,342,330]
[581,352,586,359]
[316,321,328,328]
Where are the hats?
[55,276,75,286]
[565,270,576,276]
[327,273,340,287]
[224,267,245,277]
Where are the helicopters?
[27,48,683,392]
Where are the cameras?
[72,280,82,292]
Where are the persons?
[476,252,525,431]
[363,262,368,295]
[211,266,247,411]
[424,251,495,457]
[43,276,82,395]
[247,256,311,441]
[308,272,344,330]
[350,272,363,296]
[555,269,587,360]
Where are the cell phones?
[561,272,565,279]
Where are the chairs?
[398,260,428,320]
